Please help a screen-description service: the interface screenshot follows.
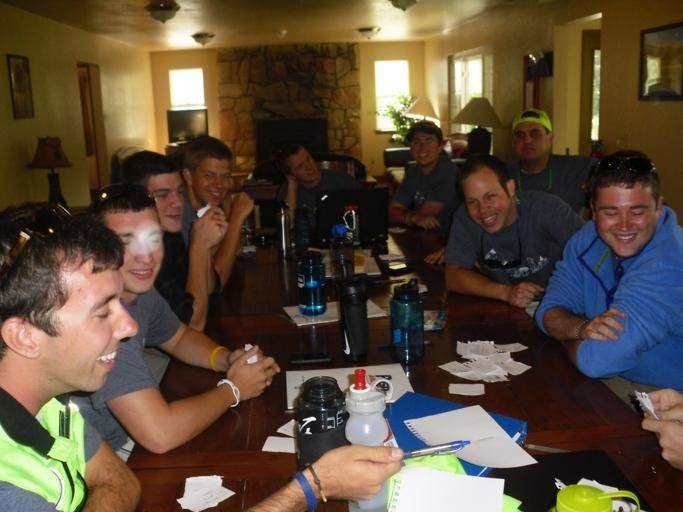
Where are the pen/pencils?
[403,440,470,458]
[523,444,571,453]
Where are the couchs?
[384,138,516,198]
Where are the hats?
[511,109,552,132]
[405,120,442,140]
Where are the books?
[283,298,389,327]
[286,363,415,412]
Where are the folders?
[383,391,527,478]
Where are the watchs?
[218,379,241,407]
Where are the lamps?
[356,28,373,37]
[144,0,180,24]
[449,97,505,159]
[388,1,417,11]
[191,32,215,46]
[26,134,72,210]
[401,95,442,122]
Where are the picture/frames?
[637,20,682,103]
[4,53,34,121]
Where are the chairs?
[272,153,366,223]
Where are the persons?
[57,185,281,463]
[534,151,683,416]
[271,143,365,222]
[640,389,682,469]
[443,155,584,308]
[390,122,465,244]
[118,152,228,332]
[1,211,401,511]
[179,135,256,288]
[423,108,600,267]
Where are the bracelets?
[292,471,319,512]
[211,347,226,371]
[306,461,327,502]
[573,321,586,340]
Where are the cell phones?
[288,352,333,364]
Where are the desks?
[123,222,682,511]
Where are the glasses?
[0,202,72,287]
[596,155,656,175]
[480,230,521,269]
[93,182,155,214]
